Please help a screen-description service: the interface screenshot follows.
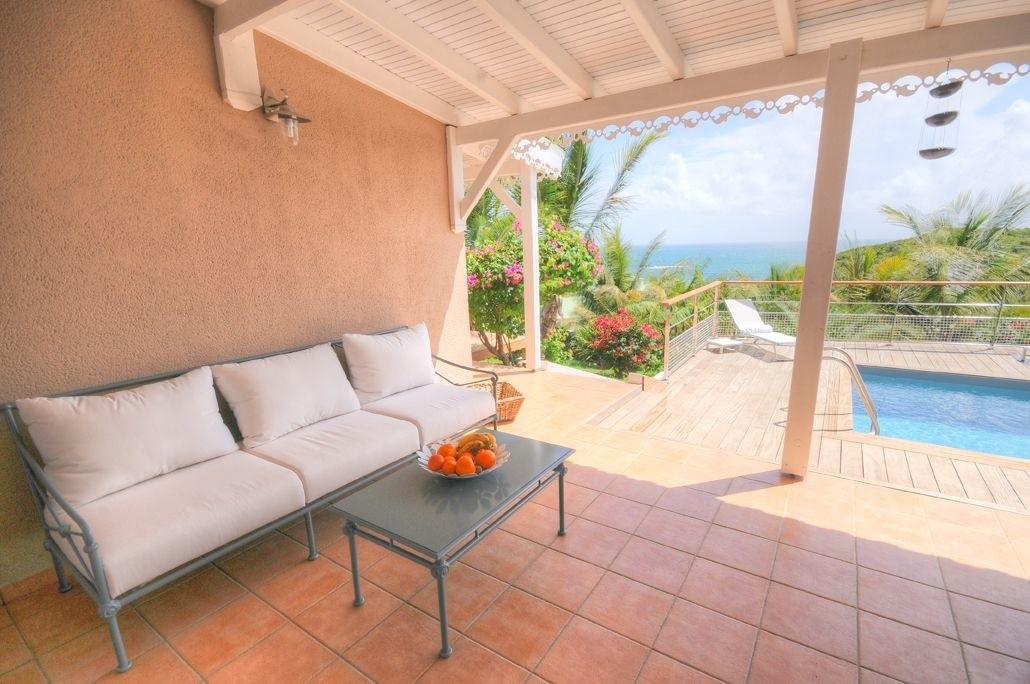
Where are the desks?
[706,338,743,353]
[324,428,576,658]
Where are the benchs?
[0,322,499,671]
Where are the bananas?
[457,432,497,455]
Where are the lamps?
[263,83,312,146]
[920,74,963,160]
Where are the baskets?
[466,382,526,427]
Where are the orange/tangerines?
[428,443,496,475]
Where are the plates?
[414,436,511,479]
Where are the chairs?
[724,298,796,361]
[1019,346,1030,362]
